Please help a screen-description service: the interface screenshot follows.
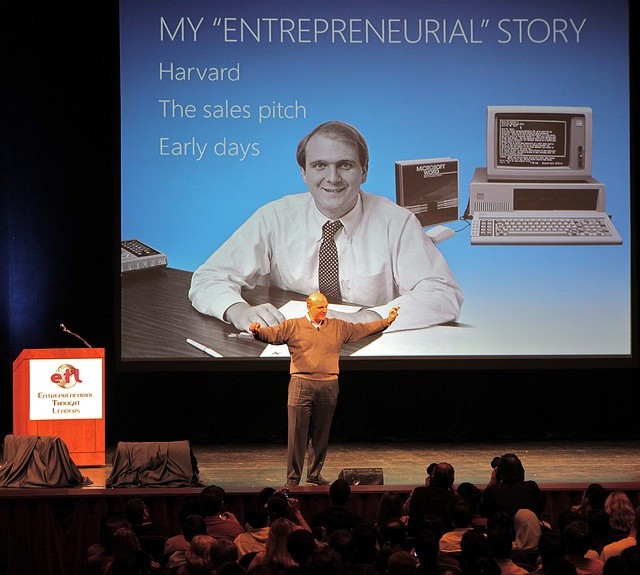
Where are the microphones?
[60,321,94,346]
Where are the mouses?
[426,222,457,244]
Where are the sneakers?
[306,478,332,486]
[285,480,298,487]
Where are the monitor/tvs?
[485,104,594,181]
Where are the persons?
[187,118,465,337]
[249,292,400,486]
[84,454,640,574]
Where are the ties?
[318,220,344,304]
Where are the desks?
[118,268,471,357]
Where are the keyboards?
[465,213,624,246]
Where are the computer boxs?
[467,167,607,211]
